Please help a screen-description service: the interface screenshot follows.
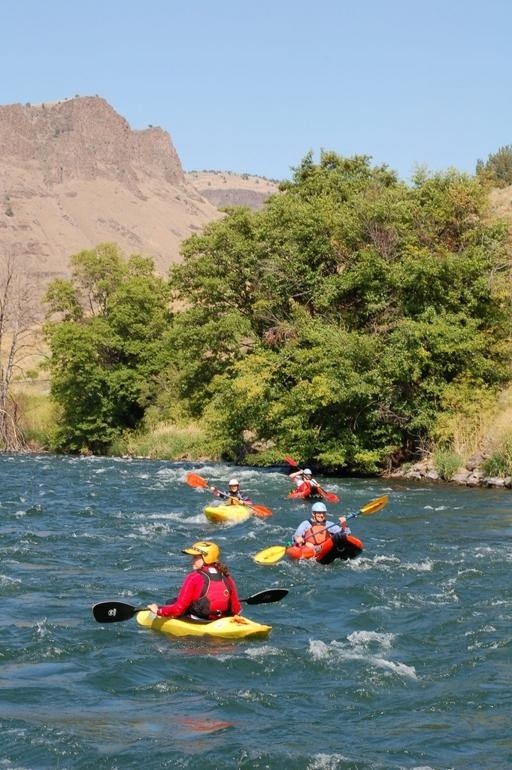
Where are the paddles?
[284,456,338,502]
[189,471,272,518]
[255,495,389,563]
[93,589,288,623]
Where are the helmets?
[228,478,238,485]
[311,502,327,512]
[303,468,312,475]
[181,541,221,565]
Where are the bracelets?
[157,607,160,615]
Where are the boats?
[135,605,275,641]
[287,488,342,505]
[200,502,254,525]
[286,532,365,566]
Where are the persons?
[146,540,243,621]
[210,479,254,505]
[293,502,351,547]
[289,468,320,489]
